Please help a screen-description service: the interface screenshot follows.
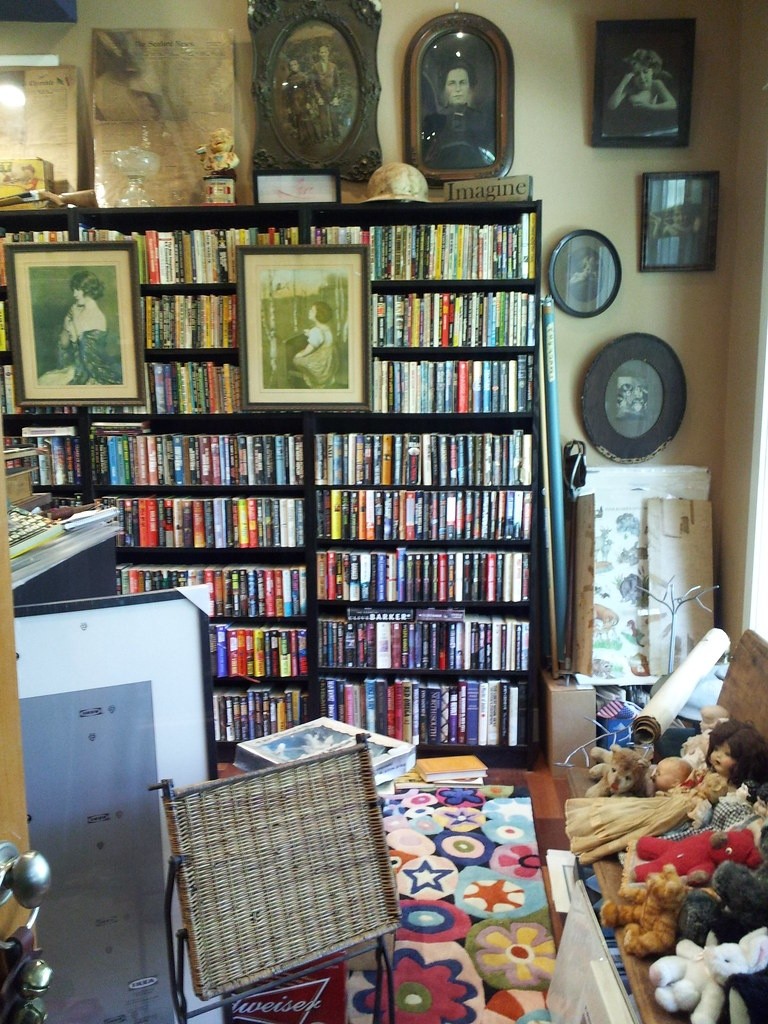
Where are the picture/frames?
[639,170,721,273]
[233,245,374,413]
[547,228,622,318]
[589,17,696,149]
[2,240,147,407]
[11,583,238,1024]
[581,331,687,466]
[401,12,517,190]
[246,0,383,183]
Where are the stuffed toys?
[581,709,768,1024]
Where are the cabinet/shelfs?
[0,198,544,773]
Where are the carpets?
[343,782,558,1024]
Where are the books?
[251,225,370,245]
[0,231,23,415]
[208,590,537,751]
[135,228,246,415]
[24,224,133,244]
[3,423,534,589]
[368,213,538,416]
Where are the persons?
[193,127,241,180]
[425,64,497,169]
[290,301,338,392]
[285,43,340,151]
[649,200,694,237]
[607,49,677,112]
[35,265,115,386]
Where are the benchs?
[566,629,768,1024]
